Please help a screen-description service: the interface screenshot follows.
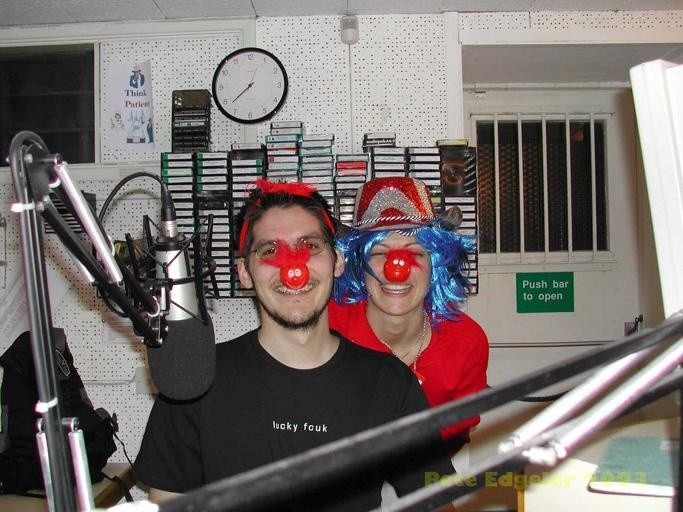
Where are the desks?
[0,461,136,512]
[514,414,683,511]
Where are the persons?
[129,64,145,88]
[325,176,489,457]
[130,178,458,511]
[145,118,153,144]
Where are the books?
[587,434,679,498]
[158,106,478,299]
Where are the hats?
[352,177,437,230]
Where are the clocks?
[211,47,289,124]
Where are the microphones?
[144,191,217,401]
